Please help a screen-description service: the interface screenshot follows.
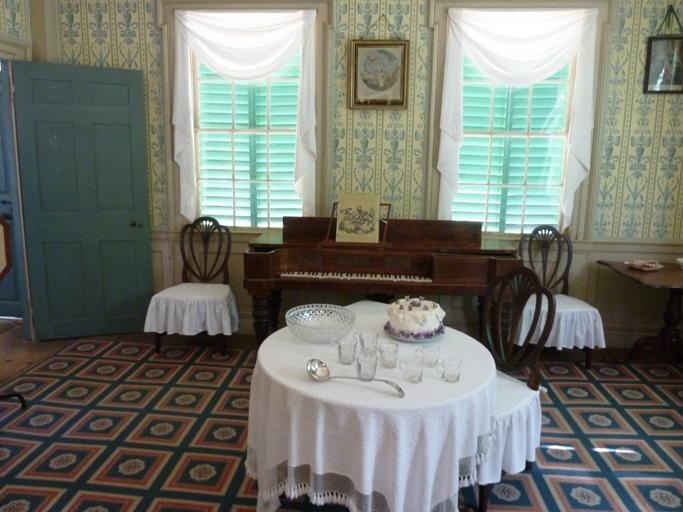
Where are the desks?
[599,260,683,364]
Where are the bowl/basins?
[676,258,683,269]
[285,304,355,345]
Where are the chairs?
[476,267,556,511]
[142,217,239,355]
[507,226,604,368]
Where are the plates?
[384,325,445,343]
[624,262,664,271]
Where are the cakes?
[382,296,446,341]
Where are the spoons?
[305,359,405,397]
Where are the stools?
[343,299,390,311]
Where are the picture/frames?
[644,33,683,94]
[347,39,407,110]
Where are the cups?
[437,358,462,383]
[353,352,378,383]
[378,341,399,369]
[331,336,357,365]
[353,329,379,357]
[400,358,424,384]
[415,346,441,368]
